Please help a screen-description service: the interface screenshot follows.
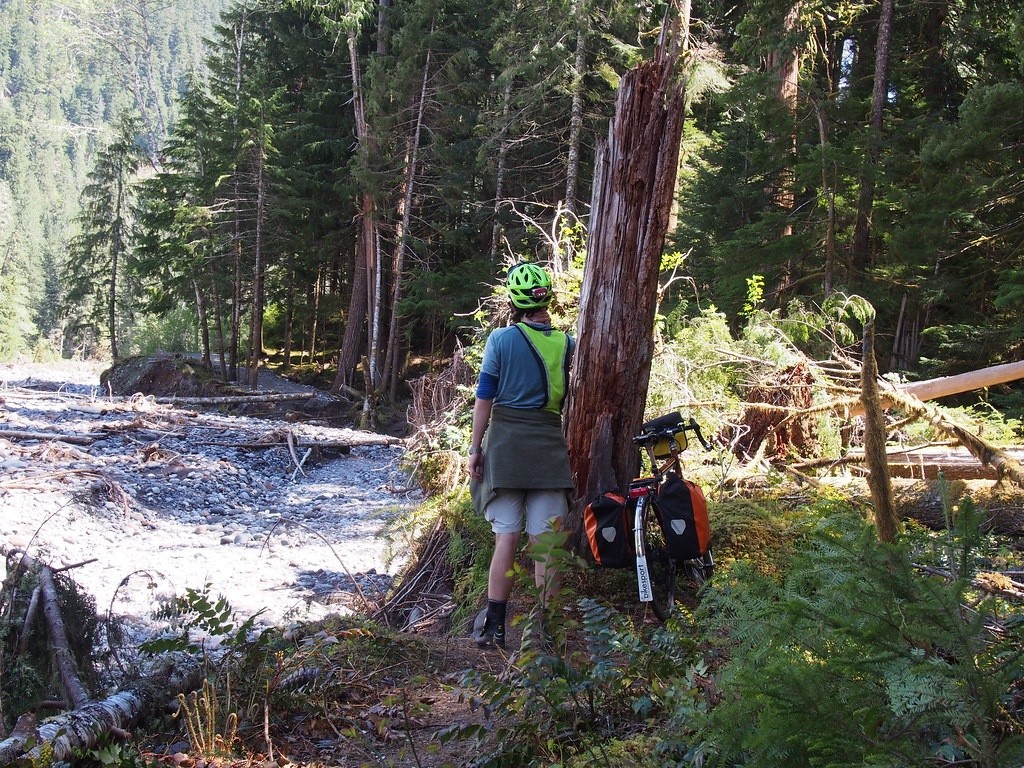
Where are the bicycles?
[624,417,710,621]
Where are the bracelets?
[469,447,482,455]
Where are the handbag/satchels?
[583,487,634,570]
[648,470,711,561]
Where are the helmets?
[504,260,553,309]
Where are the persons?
[468,264,575,650]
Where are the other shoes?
[472,612,506,650]
[539,623,567,658]
